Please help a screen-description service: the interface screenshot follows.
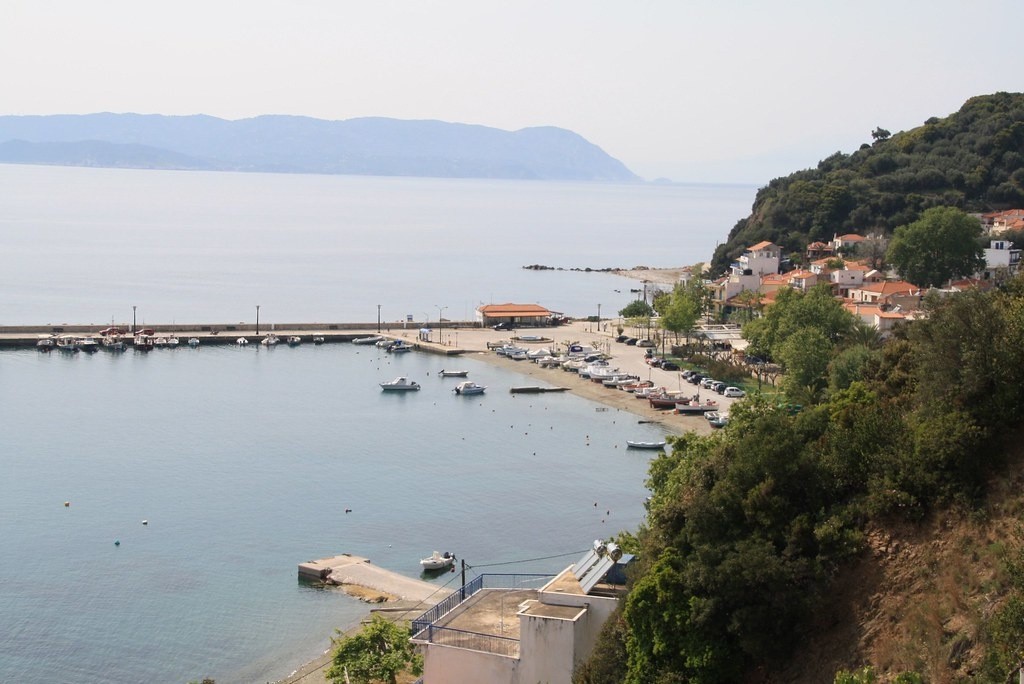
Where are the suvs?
[495,323,514,331]
[715,384,732,395]
[680,370,701,378]
[687,374,710,385]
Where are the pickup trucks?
[99,328,126,335]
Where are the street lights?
[376,304,383,332]
[255,305,261,334]
[131,306,138,334]
[435,304,449,343]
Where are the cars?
[615,335,655,347]
[724,386,746,397]
[646,356,663,363]
[136,328,154,336]
[649,358,670,367]
[701,378,714,389]
[711,382,724,391]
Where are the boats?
[261,336,280,346]
[313,337,323,344]
[56,335,78,351]
[155,338,167,348]
[168,321,179,348]
[76,334,99,351]
[352,336,382,344]
[419,550,455,570]
[236,337,249,345]
[188,338,200,346]
[133,334,153,353]
[380,377,420,391]
[388,345,414,353]
[439,369,470,376]
[627,440,666,448]
[512,349,730,428]
[102,332,126,350]
[287,336,301,347]
[374,341,395,346]
[37,339,54,351]
[455,382,487,394]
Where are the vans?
[660,362,680,370]
